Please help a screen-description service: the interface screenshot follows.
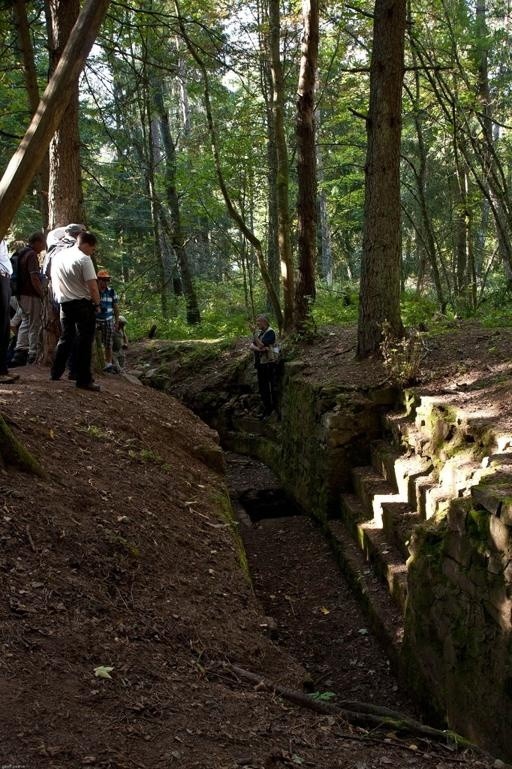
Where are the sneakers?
[108,365,113,372]
[50,371,100,391]
[257,411,272,419]
[0,372,20,382]
[10,351,35,367]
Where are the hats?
[98,270,112,278]
[119,316,129,324]
[64,223,81,233]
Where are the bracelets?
[252,331,256,335]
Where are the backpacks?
[10,248,32,295]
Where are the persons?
[247,314,286,423]
[0,222,131,391]
